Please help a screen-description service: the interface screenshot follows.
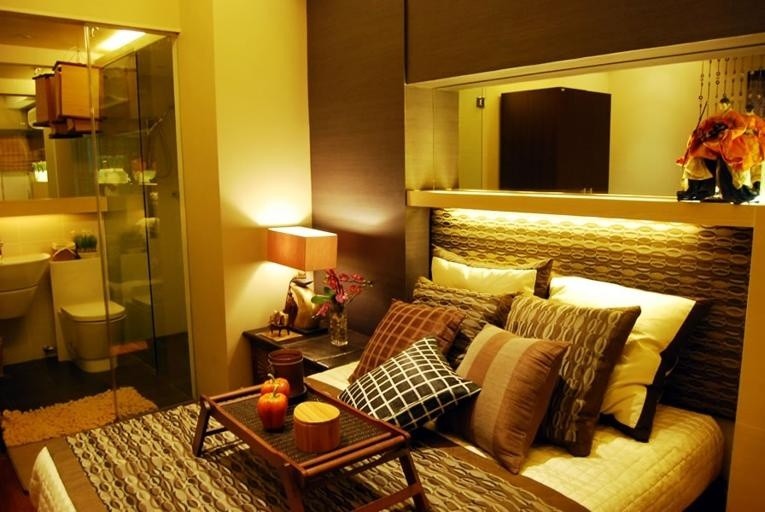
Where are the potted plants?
[31,160,48,182]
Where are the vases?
[329,312,349,347]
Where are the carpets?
[1,386,159,446]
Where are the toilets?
[48,254,127,373]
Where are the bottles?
[293,400,341,452]
[73,229,97,252]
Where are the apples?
[257,385,291,431]
[260,373,290,397]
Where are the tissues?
[52,241,77,261]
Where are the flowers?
[310,269,376,320]
[71,231,98,252]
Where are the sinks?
[0,252,51,320]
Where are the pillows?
[339,336,483,454]
[411,276,525,373]
[504,289,641,457]
[429,245,553,299]
[435,322,572,473]
[542,270,713,443]
[347,297,467,384]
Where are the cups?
[267,348,304,396]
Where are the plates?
[286,386,308,403]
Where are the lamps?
[266,226,338,338]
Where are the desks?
[192,382,434,512]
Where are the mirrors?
[0,78,110,216]
[432,43,765,205]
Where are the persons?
[741,103,764,195]
[676,97,747,203]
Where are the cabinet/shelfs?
[500,88,611,194]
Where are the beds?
[27,359,725,511]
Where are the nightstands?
[241,324,371,384]
